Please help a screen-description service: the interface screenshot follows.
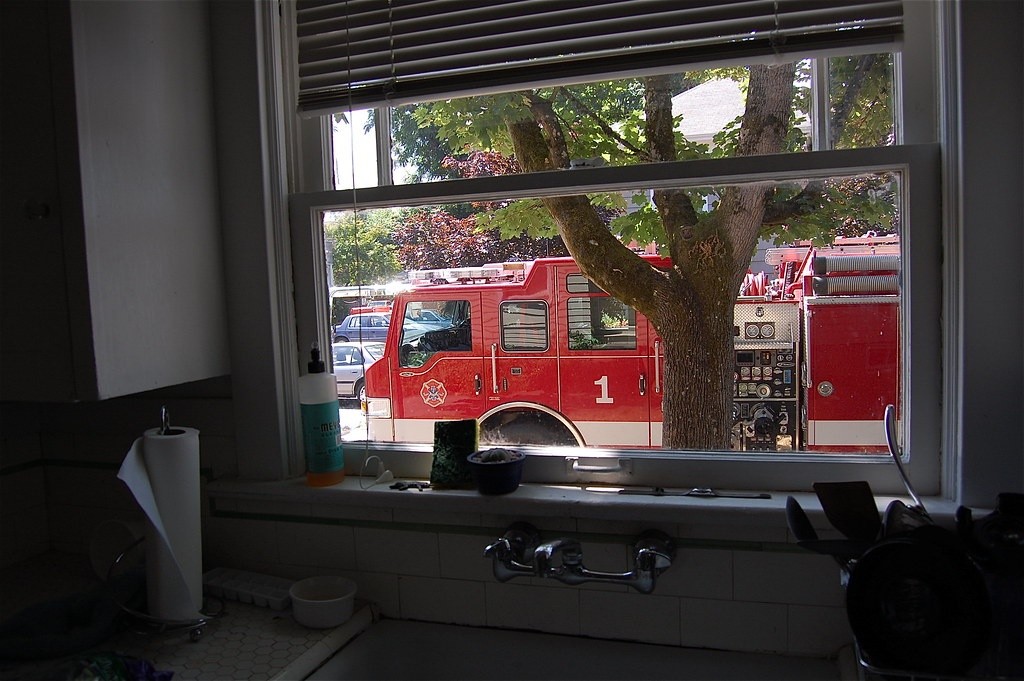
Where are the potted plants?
[467,447,526,495]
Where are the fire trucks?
[359,246,907,453]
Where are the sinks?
[301,618,855,679]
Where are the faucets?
[534,534,584,586]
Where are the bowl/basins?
[289,576,356,629]
[89,521,144,581]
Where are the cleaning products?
[296,338,347,490]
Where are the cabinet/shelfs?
[0,0,235,403]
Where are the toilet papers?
[116,425,212,621]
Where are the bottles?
[297,348,344,486]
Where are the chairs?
[334,354,348,365]
[351,351,361,364]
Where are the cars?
[407,307,453,329]
[332,341,388,404]
[335,310,426,346]
